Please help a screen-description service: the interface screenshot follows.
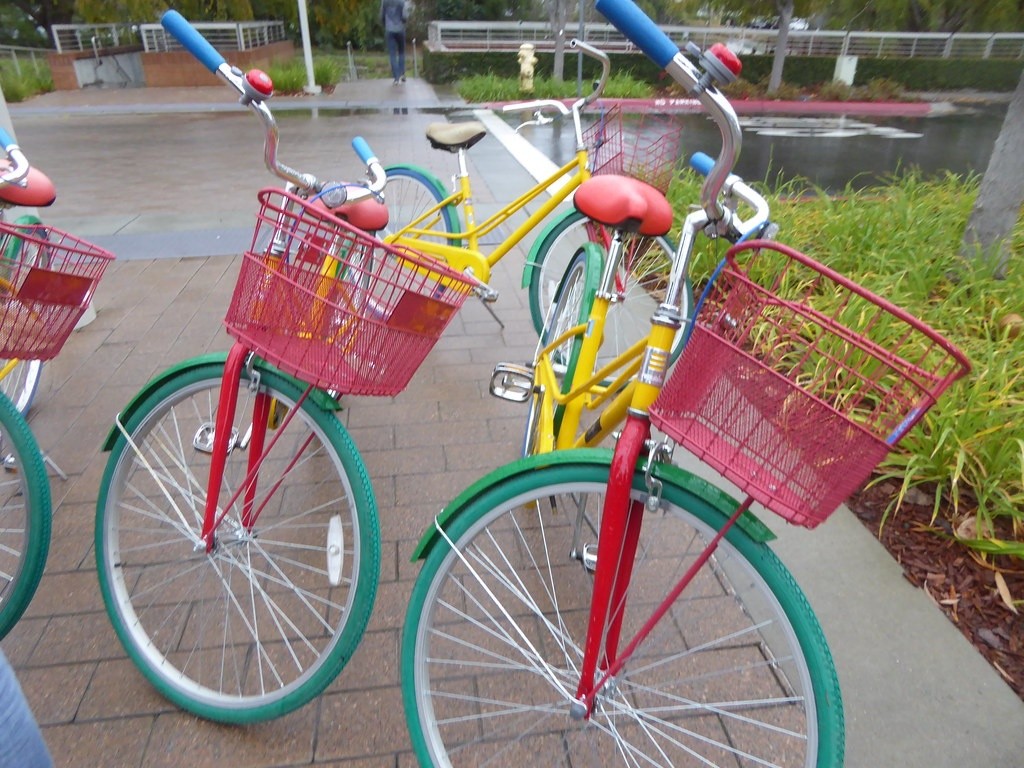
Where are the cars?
[745,16,823,57]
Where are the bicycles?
[349,35,692,393]
[1,131,116,635]
[94,10,480,726]
[400,0,977,768]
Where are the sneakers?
[401,76,406,82]
[393,80,398,86]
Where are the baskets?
[0,221,116,361]
[647,240,972,532]
[584,99,680,199]
[221,189,478,398]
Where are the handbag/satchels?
[402,1,415,21]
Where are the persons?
[381,0,417,86]
